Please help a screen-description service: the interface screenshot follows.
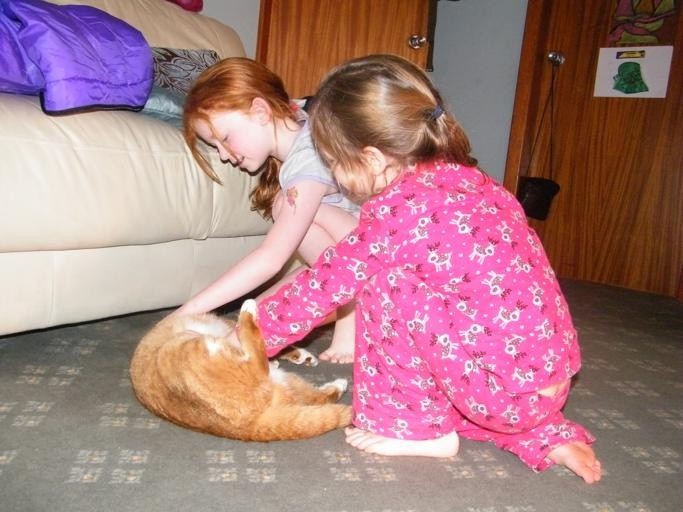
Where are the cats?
[128,296,355,445]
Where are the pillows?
[140,47,221,125]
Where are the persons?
[216,56,604,489]
[151,57,365,367]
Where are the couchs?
[0,0,304,337]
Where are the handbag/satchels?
[518,176,560,221]
